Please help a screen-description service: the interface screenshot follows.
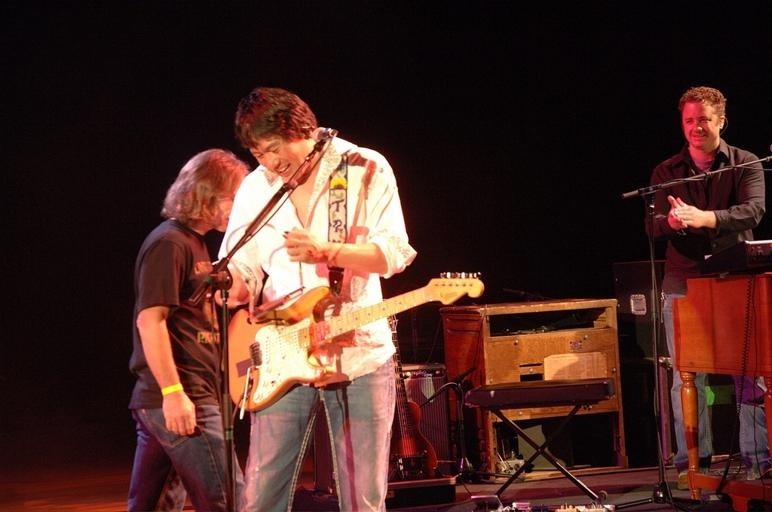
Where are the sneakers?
[746,464,772,480]
[677,469,707,490]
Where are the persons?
[111,148,255,510]
[640,84,772,491]
[200,86,421,512]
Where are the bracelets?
[161,383,184,399]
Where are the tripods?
[612,160,706,512]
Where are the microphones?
[310,126,340,139]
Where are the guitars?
[383,299,438,482]
[228,272,485,413]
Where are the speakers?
[313,361,458,478]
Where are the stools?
[467,378,615,504]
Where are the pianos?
[672,272,772,500]
